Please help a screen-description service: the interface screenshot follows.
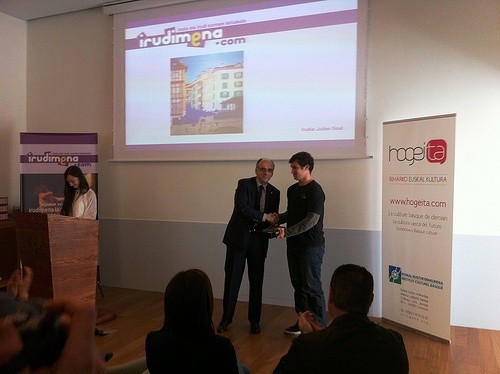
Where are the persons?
[271,154,327,334]
[60,165,98,222]
[213,157,281,336]
[269,262,409,374]
[143,268,252,374]
[1,259,116,374]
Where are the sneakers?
[285,318,301,335]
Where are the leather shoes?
[250,321,261,334]
[217,320,233,332]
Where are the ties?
[254,185,264,212]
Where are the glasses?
[255,167,274,173]
